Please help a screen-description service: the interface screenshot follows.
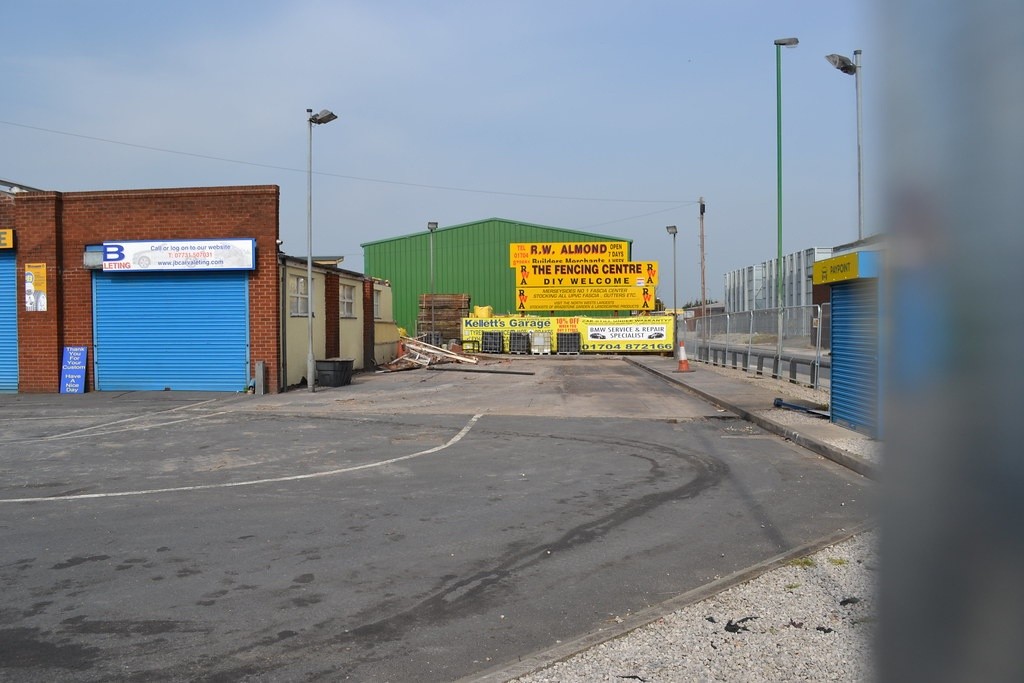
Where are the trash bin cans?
[316,360,356,387]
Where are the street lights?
[671,341,697,373]
[305,108,338,394]
[667,225,678,350]
[427,221,439,348]
[823,48,863,241]
[773,37,800,359]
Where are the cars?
[590,333,606,339]
[648,332,663,339]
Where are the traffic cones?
[397,340,405,359]
